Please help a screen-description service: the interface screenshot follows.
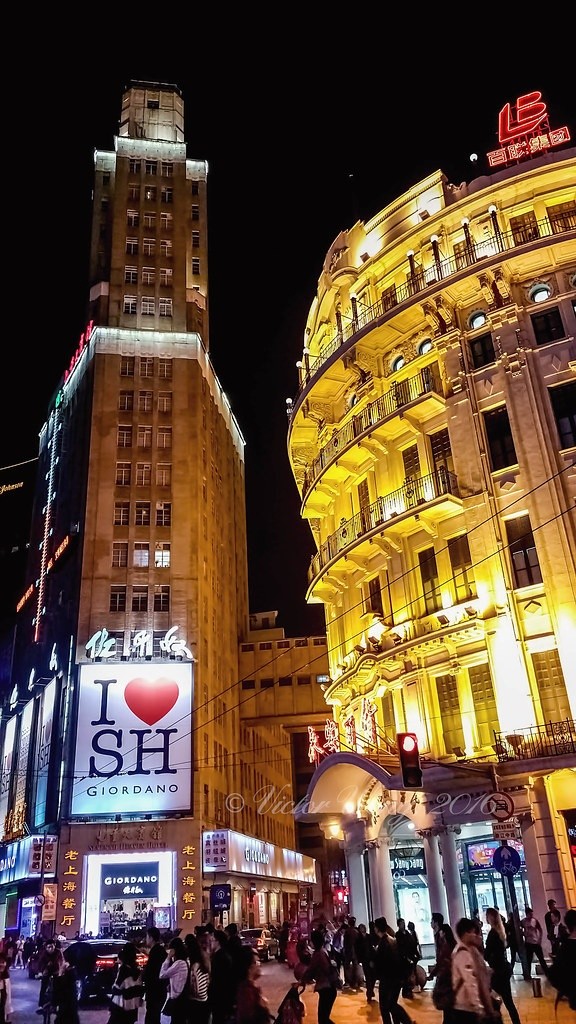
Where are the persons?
[0,898,576,1024]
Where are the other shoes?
[412,985,421,993]
[352,986,363,992]
[513,1018,521,1024]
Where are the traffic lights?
[395,732,425,790]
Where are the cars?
[240,929,281,963]
[60,937,149,1008]
[27,935,78,980]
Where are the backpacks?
[431,947,469,1010]
